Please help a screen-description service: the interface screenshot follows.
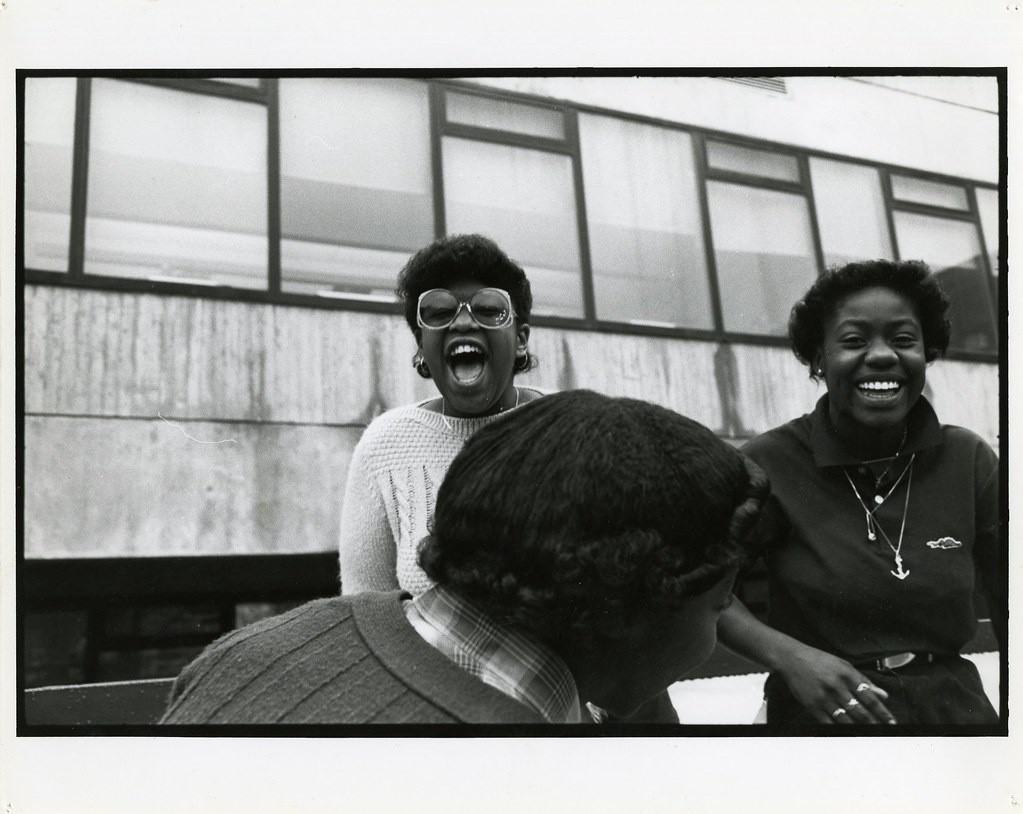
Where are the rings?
[846,698,859,707]
[856,683,870,693]
[832,708,846,717]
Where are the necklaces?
[442,385,520,415]
[843,430,915,580]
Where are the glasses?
[416,287,520,331]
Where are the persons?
[715,258,1001,726]
[157,388,770,724]
[335,235,681,724]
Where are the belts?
[849,651,963,677]
